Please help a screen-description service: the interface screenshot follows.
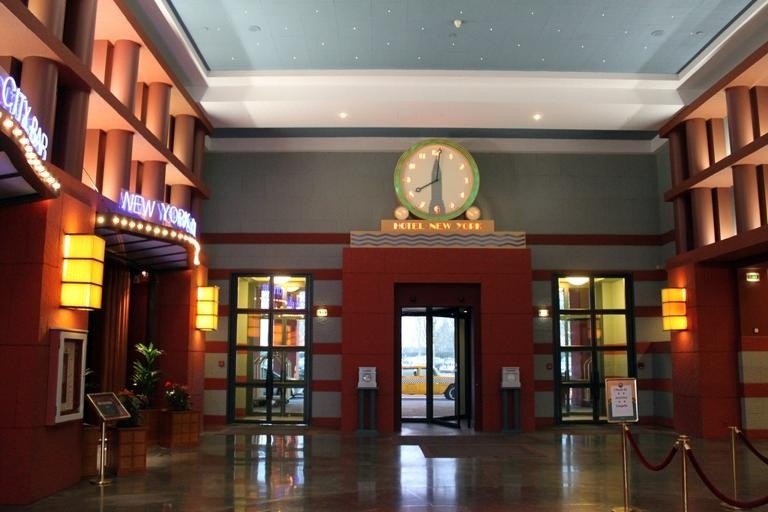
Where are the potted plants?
[133,344,168,443]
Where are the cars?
[299,351,305,379]
[260,364,303,399]
[401,364,456,401]
[559,356,572,380]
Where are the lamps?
[538,305,549,319]
[316,304,328,321]
[195,284,220,331]
[660,286,688,332]
[59,232,107,311]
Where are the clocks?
[392,137,482,220]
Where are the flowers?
[162,381,193,411]
[117,388,143,427]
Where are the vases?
[159,410,201,449]
[115,424,147,476]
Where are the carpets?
[392,434,544,443]
[418,440,547,458]
